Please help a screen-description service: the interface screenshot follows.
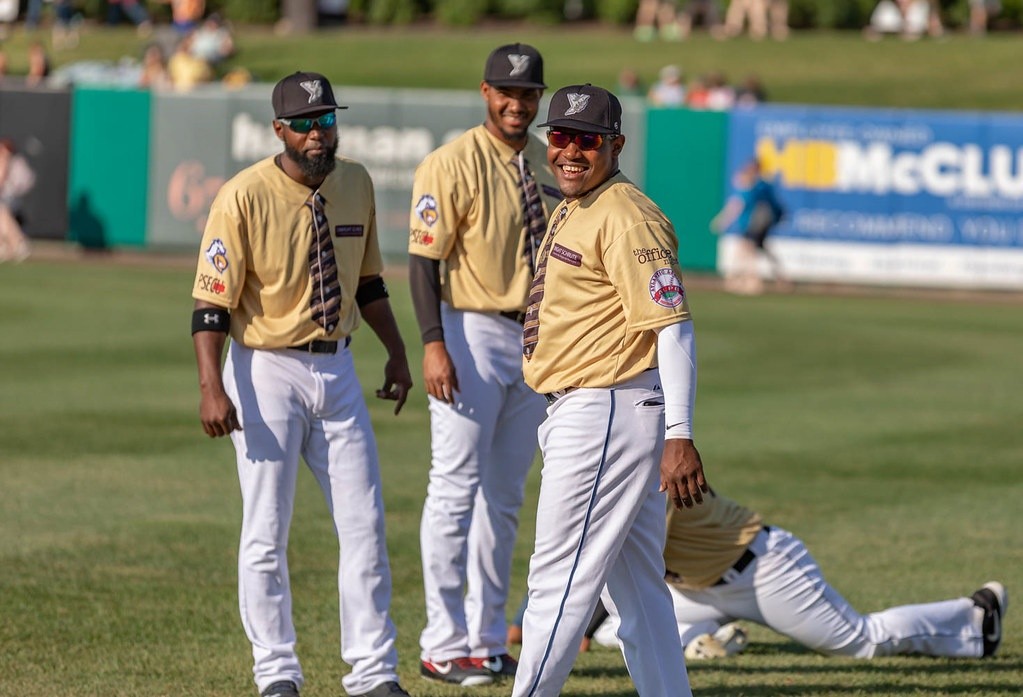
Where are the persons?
[189,70,414,696]
[508,477,1007,660]
[408,44,563,690]
[2,1,252,88]
[503,82,708,696]
[0,144,40,268]
[630,1,1002,45]
[613,62,775,108]
[708,154,794,292]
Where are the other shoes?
[261,681,300,697]
[350,681,411,697]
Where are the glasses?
[277,110,336,133]
[548,130,621,151]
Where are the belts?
[500,310,526,326]
[287,335,352,354]
[544,366,658,406]
[711,526,770,586]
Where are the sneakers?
[469,652,519,675]
[685,623,748,660]
[419,657,494,688]
[971,581,1009,659]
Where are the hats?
[272,71,349,119]
[537,83,622,134]
[485,43,548,89]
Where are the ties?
[511,152,547,279]
[522,205,568,362]
[305,193,343,338]
